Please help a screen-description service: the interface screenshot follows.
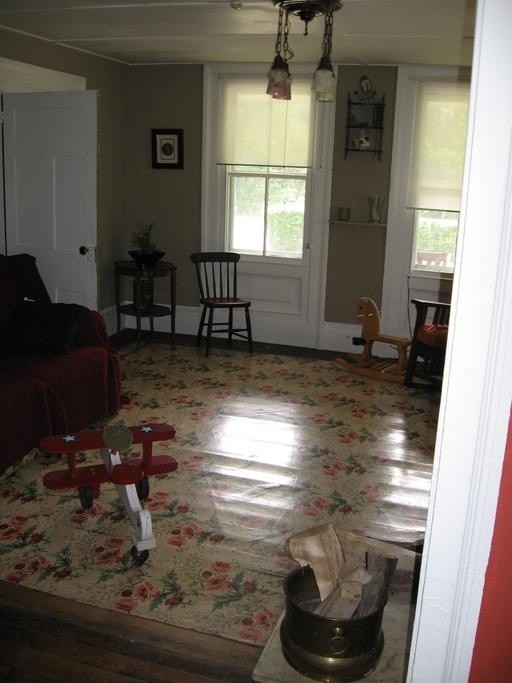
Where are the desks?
[114,258,181,350]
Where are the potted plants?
[126,218,167,265]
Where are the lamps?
[264,1,344,104]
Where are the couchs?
[0,252,124,478]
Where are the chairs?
[403,298,450,389]
[190,251,254,356]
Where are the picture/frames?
[150,126,185,171]
